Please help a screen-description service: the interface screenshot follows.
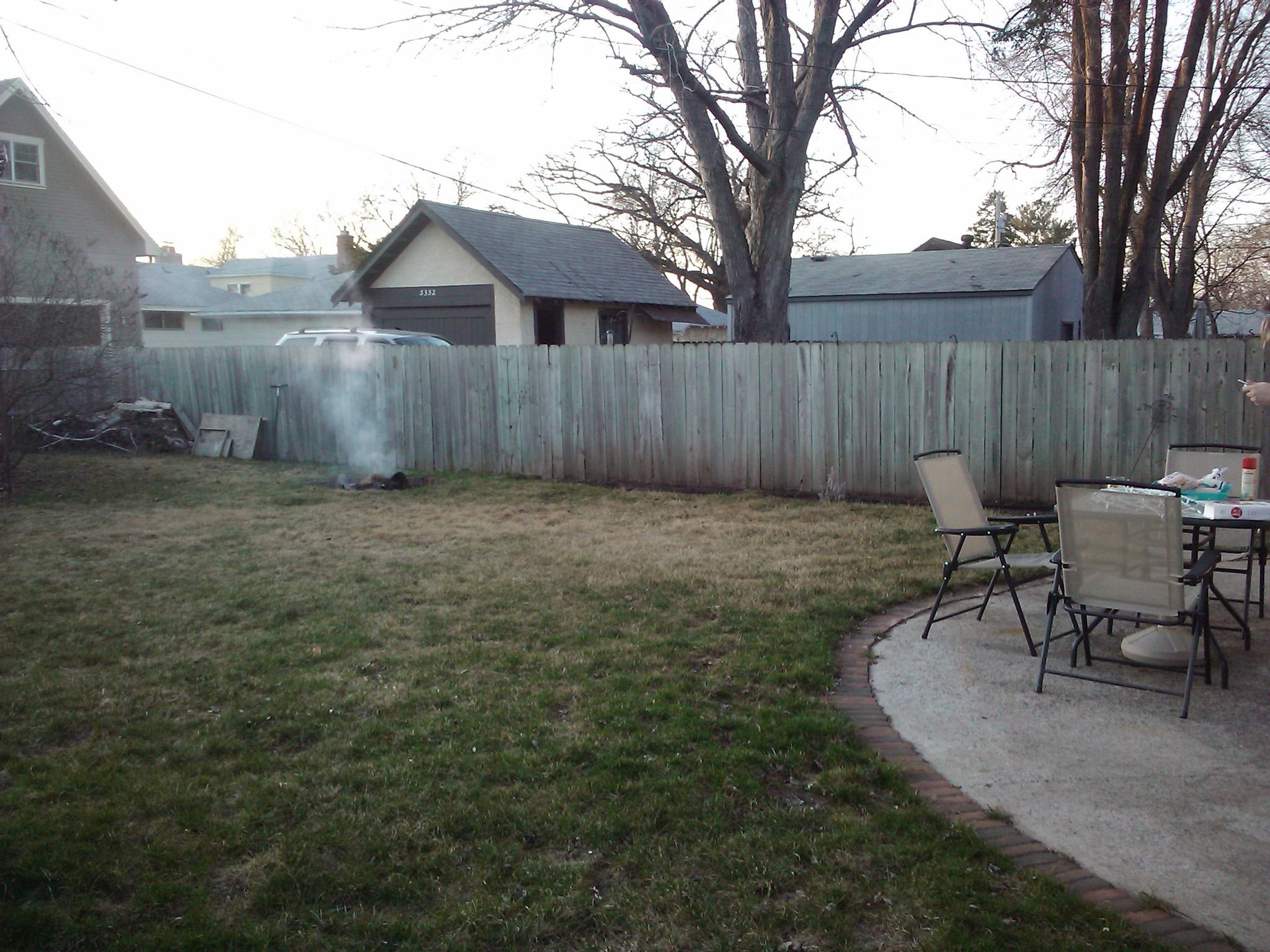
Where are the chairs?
[914,444,1261,718]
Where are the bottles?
[1240,457,1257,501]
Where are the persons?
[1242,315,1270,406]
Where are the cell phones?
[1237,379,1249,385]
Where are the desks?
[1070,499,1270,690]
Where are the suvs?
[276,326,457,347]
